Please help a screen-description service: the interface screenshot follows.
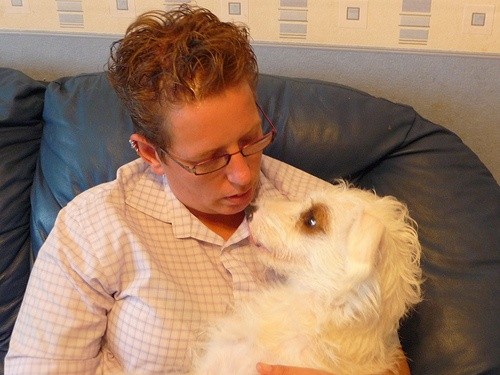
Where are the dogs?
[186,176,433,374]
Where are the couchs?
[0,67,500,375]
